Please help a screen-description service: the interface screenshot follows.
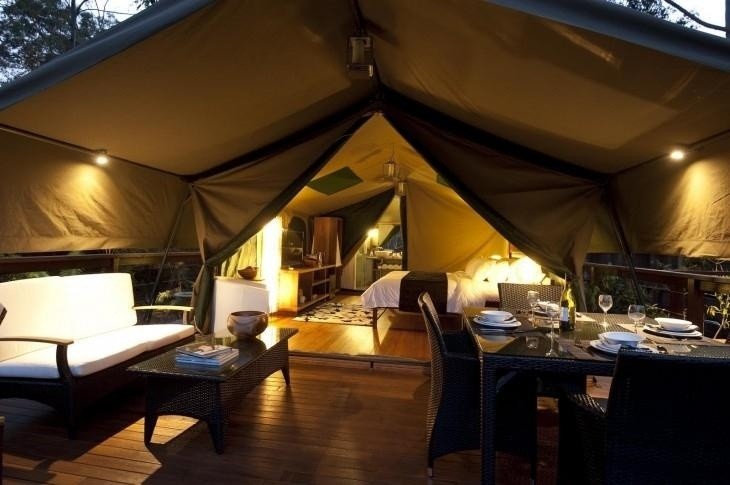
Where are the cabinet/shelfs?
[313,216,343,265]
[278,264,336,316]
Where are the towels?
[598,336,621,351]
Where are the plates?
[643,325,703,338]
[589,339,659,357]
[474,318,522,328]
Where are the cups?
[528,291,540,329]
[546,302,561,338]
[526,335,539,351]
[599,295,613,326]
[545,338,559,358]
[628,305,645,334]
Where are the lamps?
[368,228,379,256]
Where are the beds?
[372,271,500,330]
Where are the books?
[175,342,239,365]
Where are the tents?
[0,0,730,332]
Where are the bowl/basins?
[480,310,508,322]
[226,311,268,339]
[603,331,642,349]
[654,317,692,331]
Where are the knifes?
[480,328,523,333]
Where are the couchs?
[0,272,196,441]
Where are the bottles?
[559,270,576,332]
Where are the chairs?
[497,283,564,309]
[557,347,730,485]
[417,290,539,485]
